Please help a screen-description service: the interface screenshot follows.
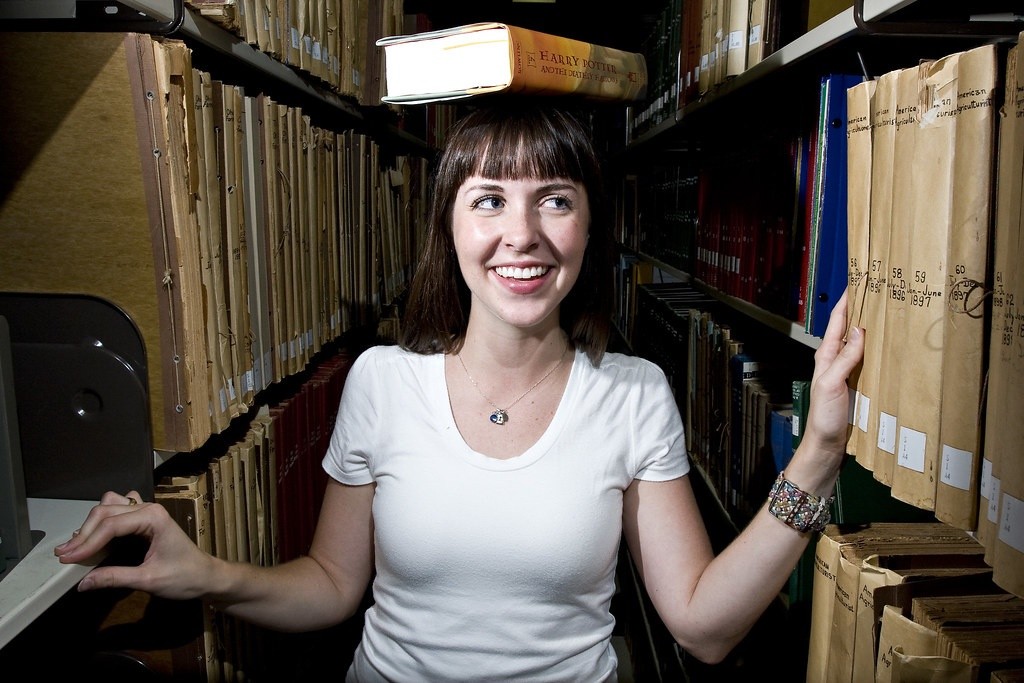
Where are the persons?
[55,95,865,683]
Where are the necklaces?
[456,344,568,425]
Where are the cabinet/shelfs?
[0,0,1023,683]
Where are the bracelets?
[768,468,836,537]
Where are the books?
[0,0,459,683]
[600,2,1024,683]
[378,21,650,104]
